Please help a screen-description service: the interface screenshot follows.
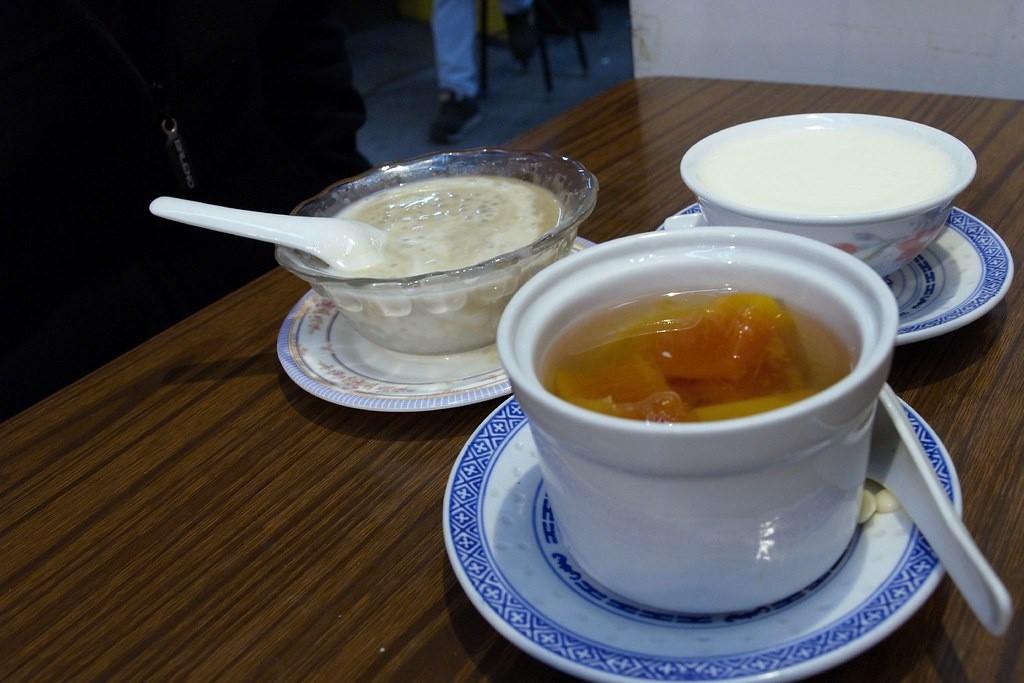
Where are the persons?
[428,0,539,144]
[0,2,374,423]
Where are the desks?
[0,76,1024,683]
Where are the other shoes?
[427,89,487,145]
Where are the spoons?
[149,196,384,273]
[866,381,1014,638]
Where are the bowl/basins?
[679,112,978,277]
[495,225,900,616]
[274,145,599,355]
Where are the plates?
[442,392,962,683]
[656,204,1014,345]
[275,233,599,413]
